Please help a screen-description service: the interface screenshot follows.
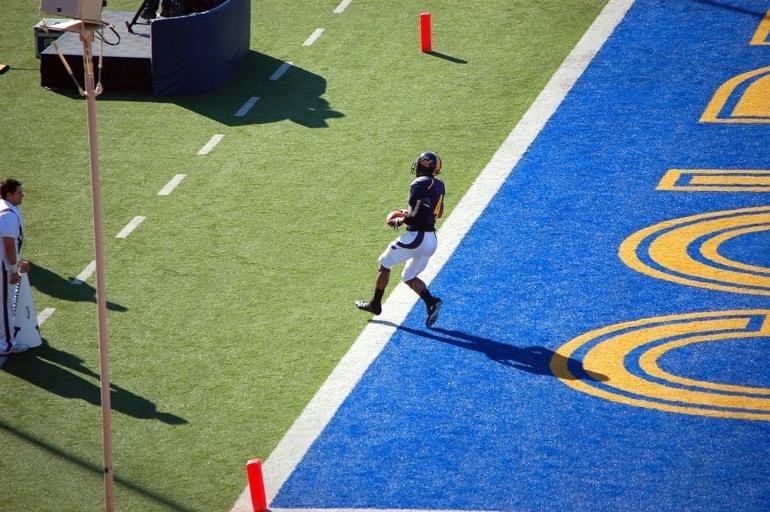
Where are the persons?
[355,151,445,328]
[0,177,30,356]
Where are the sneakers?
[0,347,29,355]
[426,298,442,327]
[356,301,382,315]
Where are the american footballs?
[386,210,406,227]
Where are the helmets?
[411,152,441,176]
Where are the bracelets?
[9,263,18,274]
